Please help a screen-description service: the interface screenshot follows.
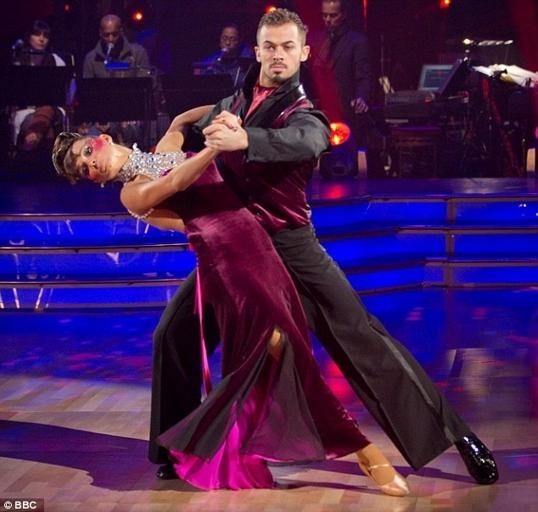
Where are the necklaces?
[110,140,174,183]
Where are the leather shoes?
[453,432,499,484]
[358,451,408,495]
[155,462,178,480]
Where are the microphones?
[217,47,230,62]
[11,39,24,50]
[103,42,115,66]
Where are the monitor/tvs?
[418,64,454,92]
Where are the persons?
[81,12,170,150]
[51,102,409,499]
[302,0,392,180]
[194,20,251,93]
[3,15,73,165]
[147,8,499,486]
